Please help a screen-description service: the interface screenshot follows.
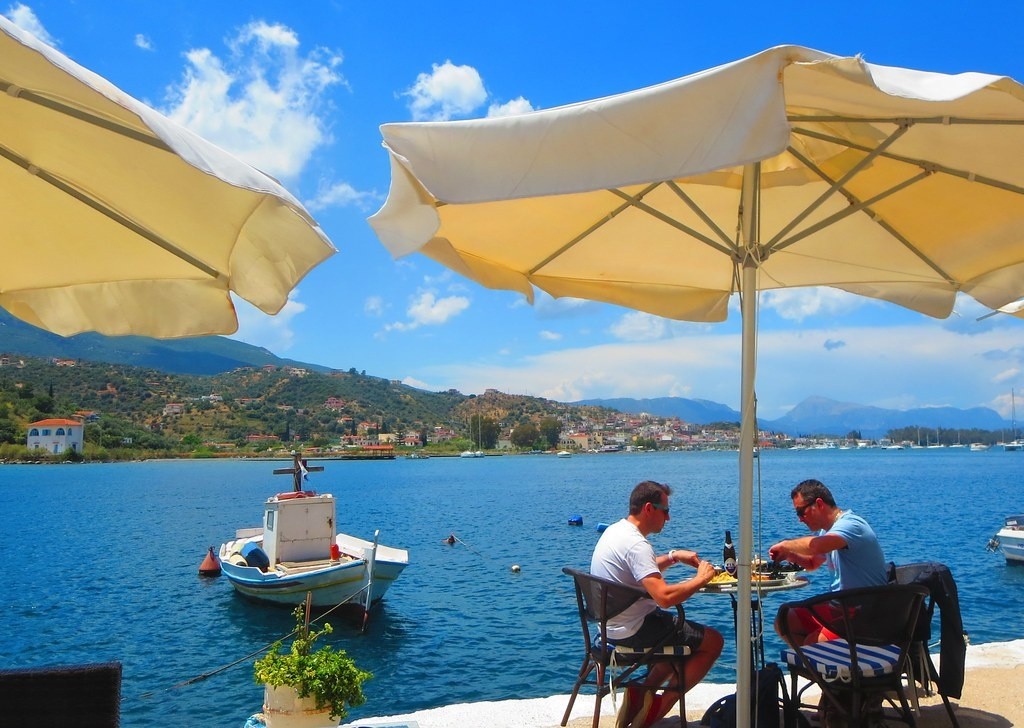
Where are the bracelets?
[668,549,679,564]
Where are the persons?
[765,478,895,727]
[586,479,725,728]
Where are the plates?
[750,578,785,587]
[751,568,798,574]
[700,578,739,585]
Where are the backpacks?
[750,662,791,728]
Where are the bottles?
[723,529,737,577]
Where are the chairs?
[560,567,691,728]
[0,662,124,728]
[772,561,959,728]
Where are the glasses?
[797,502,814,516]
[642,499,669,515]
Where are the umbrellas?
[0,6,341,346]
[368,39,1024,728]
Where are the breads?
[751,576,771,580]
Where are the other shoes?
[615,687,644,728]
[633,690,662,728]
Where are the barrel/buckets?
[568,514,582,527]
[595,522,611,531]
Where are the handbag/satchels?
[700,694,735,728]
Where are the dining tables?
[679,577,811,728]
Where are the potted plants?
[253,599,375,728]
